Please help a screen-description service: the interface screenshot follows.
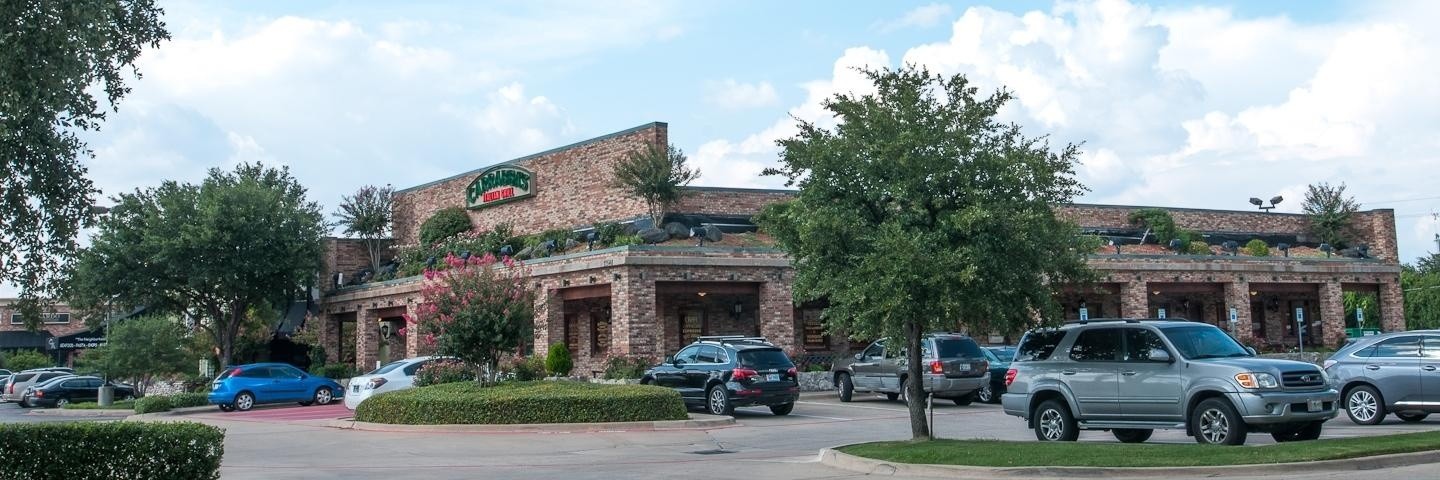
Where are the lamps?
[1107,240,1371,260]
[729,295,743,321]
[690,227,707,247]
[426,234,600,270]
[382,322,398,340]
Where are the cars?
[345,355,517,412]
[971,344,1018,404]
[1323,329,1440,426]
[207,362,345,412]
[0,366,141,409]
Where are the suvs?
[1001,316,1341,448]
[640,335,800,416]
[831,332,992,407]
